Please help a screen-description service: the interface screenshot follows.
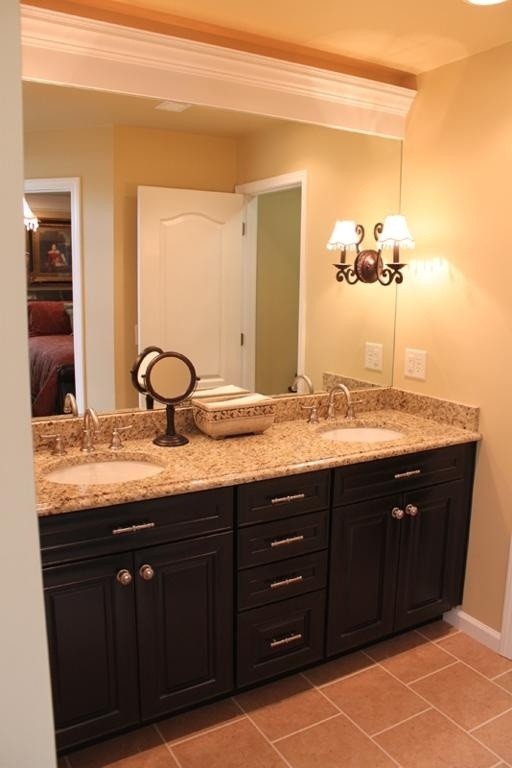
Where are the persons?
[45,242,68,268]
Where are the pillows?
[28,302,74,338]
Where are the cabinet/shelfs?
[38,488,238,761]
[325,444,472,667]
[233,469,334,691]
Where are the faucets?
[64,393,79,417]
[326,384,356,420]
[81,408,101,452]
[288,374,315,395]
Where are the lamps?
[326,219,365,286]
[362,213,413,289]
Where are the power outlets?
[403,347,428,380]
[361,341,383,374]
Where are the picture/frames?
[26,215,72,287]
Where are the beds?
[26,298,75,417]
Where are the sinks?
[314,420,410,444]
[37,453,169,484]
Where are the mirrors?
[17,73,407,429]
[139,353,201,446]
[128,345,162,412]
